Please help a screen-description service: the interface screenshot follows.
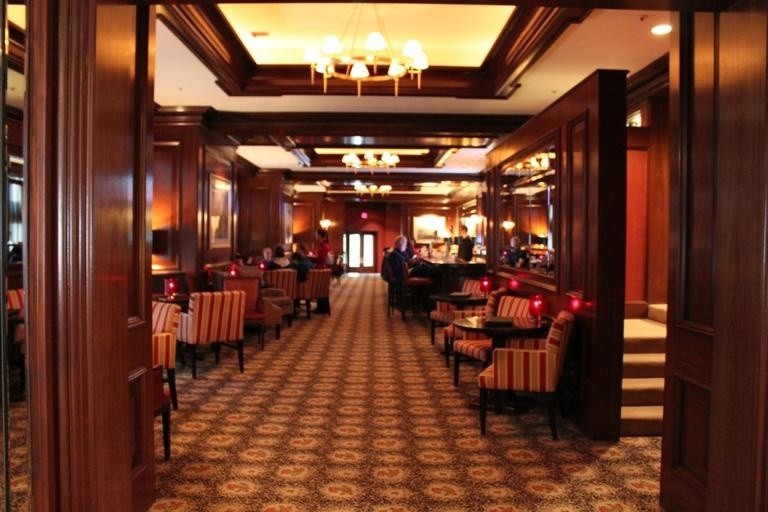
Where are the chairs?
[402,262,432,321]
[384,262,398,316]
[152,256,332,461]
[430,279,575,441]
[5,289,25,390]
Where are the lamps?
[341,153,400,175]
[354,184,392,197]
[307,4,429,98]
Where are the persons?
[268,244,290,269]
[290,251,314,282]
[313,229,330,314]
[392,235,436,289]
[504,236,529,270]
[457,224,472,261]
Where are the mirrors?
[492,129,561,291]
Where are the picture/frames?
[208,173,232,250]
